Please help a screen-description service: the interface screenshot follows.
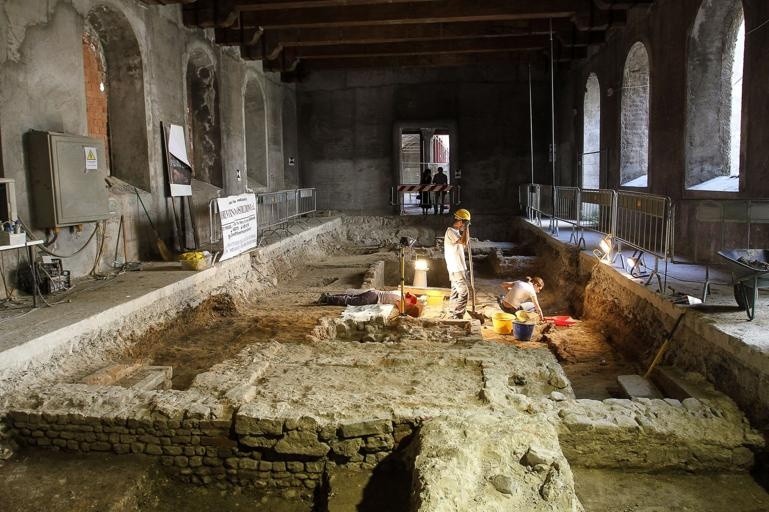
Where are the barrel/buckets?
[426,289,445,305]
[512,320,536,341]
[515,309,540,323]
[491,312,516,335]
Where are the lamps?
[412,257,430,288]
[625,256,650,278]
[599,233,614,264]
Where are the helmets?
[454,208,470,220]
[407,292,417,304]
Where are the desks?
[0,238,44,308]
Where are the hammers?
[121,215,130,269]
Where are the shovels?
[464,221,485,324]
[544,314,577,326]
[132,186,171,262]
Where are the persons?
[316,289,421,316]
[498,274,548,319]
[443,207,473,318]
[418,170,430,215]
[432,166,449,214]
